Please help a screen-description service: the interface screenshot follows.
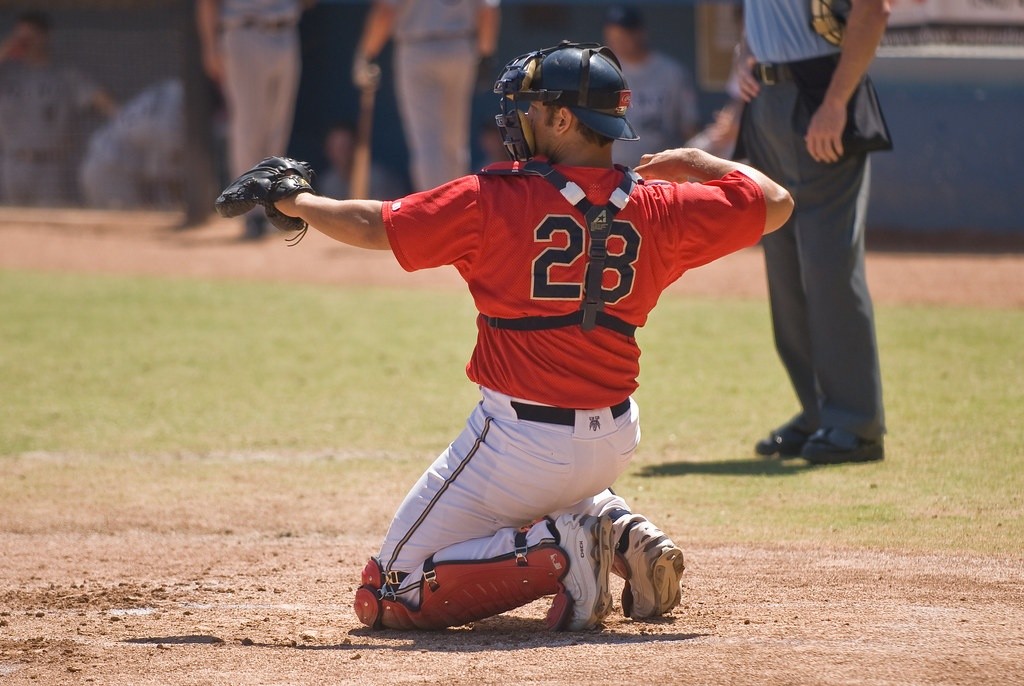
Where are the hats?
[602,3,644,31]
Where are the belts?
[750,63,793,87]
[510,396,630,426]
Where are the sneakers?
[554,511,612,633]
[618,520,684,619]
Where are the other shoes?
[755,420,812,456]
[243,207,270,239]
[801,426,885,464]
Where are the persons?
[731,0,895,461]
[215,40,795,632]
[0,0,702,240]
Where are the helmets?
[531,42,640,142]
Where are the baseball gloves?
[216,154,315,231]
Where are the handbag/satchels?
[793,52,893,184]
